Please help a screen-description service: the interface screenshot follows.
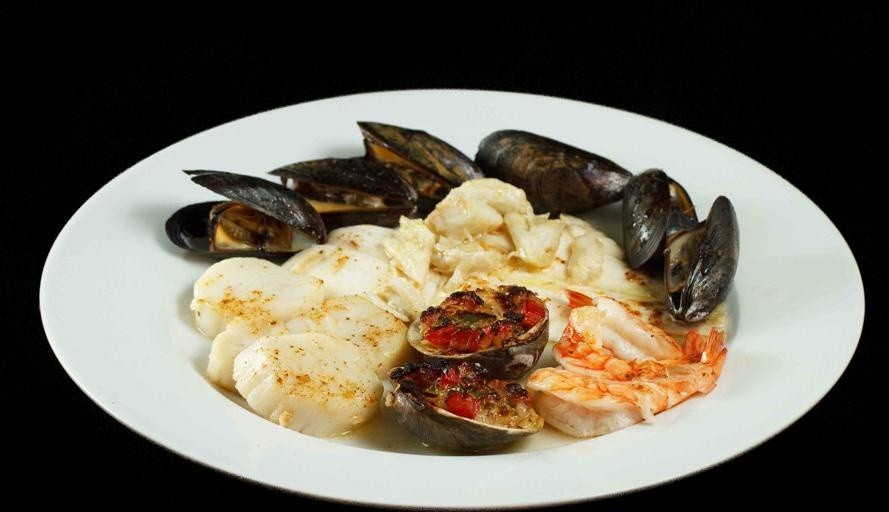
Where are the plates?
[36,85,867,507]
